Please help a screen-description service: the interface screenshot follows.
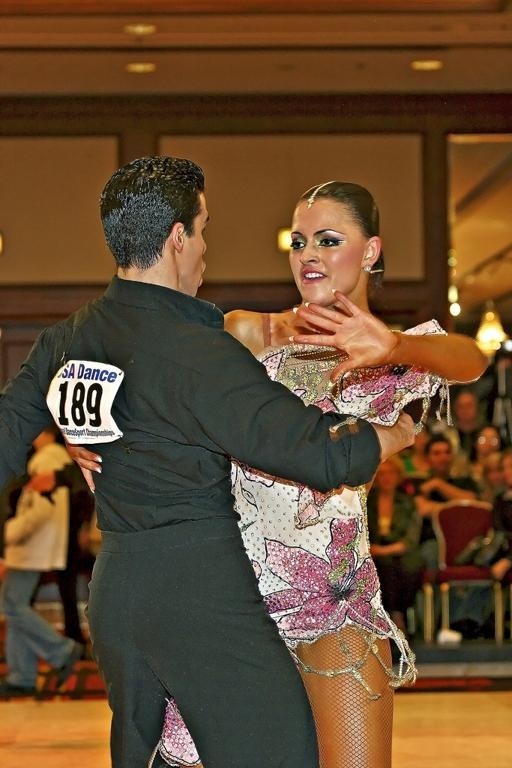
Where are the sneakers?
[0,678,39,703]
[52,638,86,688]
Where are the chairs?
[432,498,507,644]
[410,563,435,640]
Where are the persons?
[366,390,512,632]
[0,153,415,768]
[66,181,489,768]
[0,424,97,697]
